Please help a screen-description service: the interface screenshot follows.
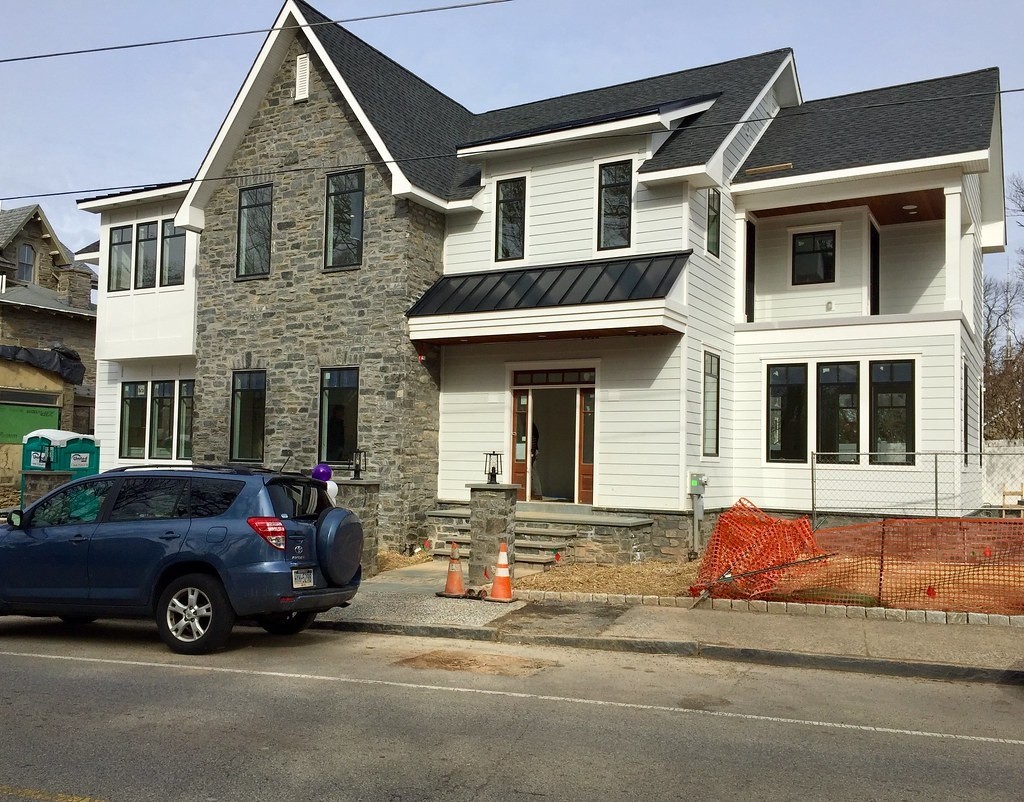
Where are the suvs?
[0,462,364,656]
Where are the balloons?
[312,464,338,505]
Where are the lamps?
[347,449,367,481]
[39,441,58,471]
[484,450,505,483]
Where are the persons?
[328,406,345,461]
[531,423,542,500]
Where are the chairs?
[1002,482,1024,518]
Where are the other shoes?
[532,495,542,500]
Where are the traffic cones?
[435,542,468,599]
[484,542,519,603]
